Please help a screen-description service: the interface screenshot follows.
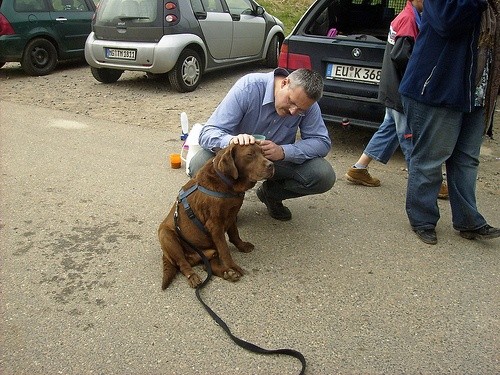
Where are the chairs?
[61,0,76,10]
[337,1,386,32]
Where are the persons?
[346,0,450,198]
[188,66,335,221]
[398,0,500,244]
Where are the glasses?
[286,85,307,117]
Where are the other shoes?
[344,165,381,187]
[460,225,500,240]
[438,181,449,199]
[414,227,438,245]
[256,185,292,222]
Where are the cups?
[253,134,266,144]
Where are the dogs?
[157,137,278,292]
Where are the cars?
[84,0,285,94]
[0,0,98,76]
[277,0,385,130]
